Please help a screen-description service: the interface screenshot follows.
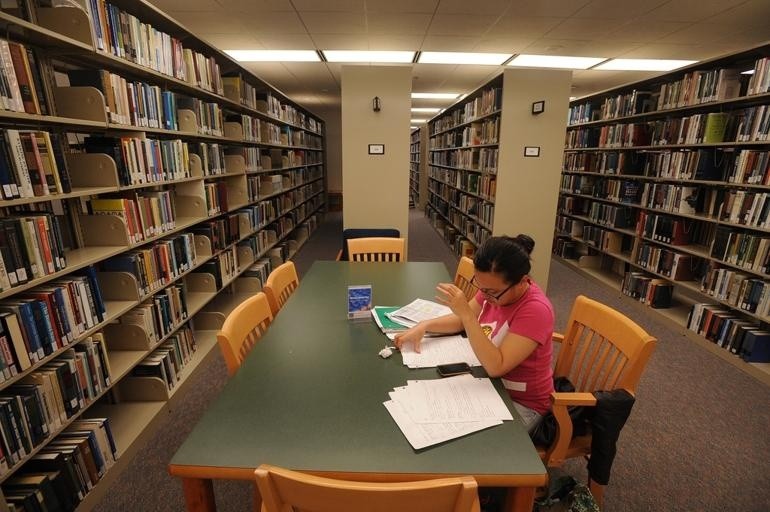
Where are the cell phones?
[435,360,473,378]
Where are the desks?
[167,260,549,512]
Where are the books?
[738,58,770,142]
[653,68,739,146]
[53,0,327,392]
[1,39,118,511]
[648,148,770,229]
[551,90,654,276]
[621,215,770,362]
[410,87,503,258]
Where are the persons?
[394,234,556,435]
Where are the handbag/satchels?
[536,467,600,512]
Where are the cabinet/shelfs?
[551,41,770,387]
[339,66,413,264]
[409,68,573,296]
[0,0,328,512]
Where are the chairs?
[535,295,657,512]
[252,464,481,512]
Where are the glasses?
[470,275,512,303]
